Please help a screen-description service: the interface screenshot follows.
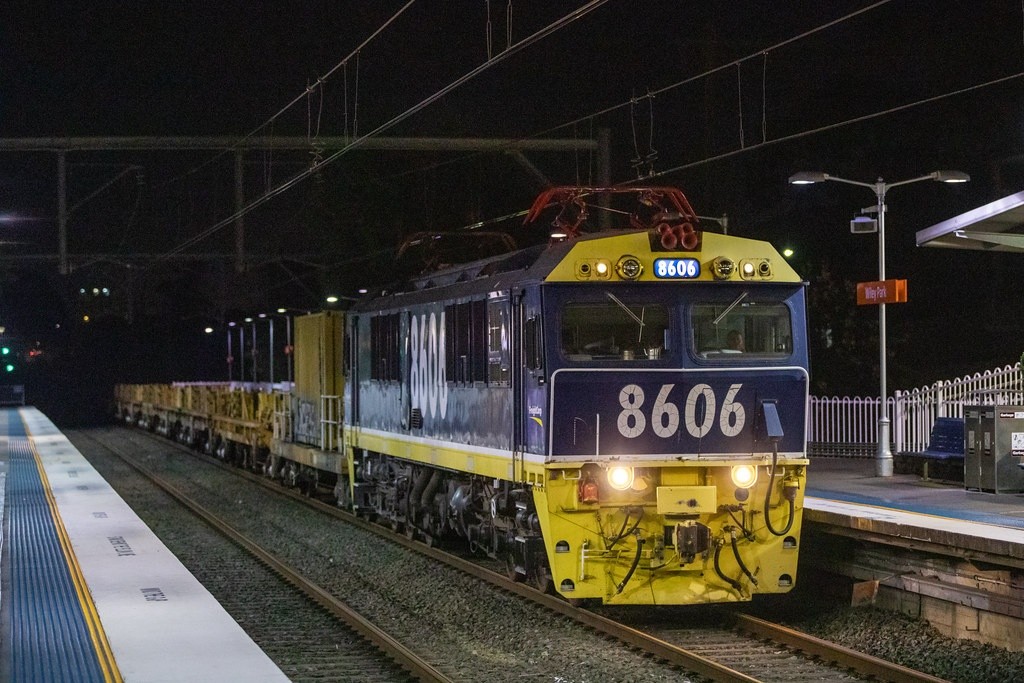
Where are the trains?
[110,207,810,604]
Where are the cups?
[623,350,635,360]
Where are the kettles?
[643,346,662,359]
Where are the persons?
[719,329,746,353]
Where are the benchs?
[900,418,966,482]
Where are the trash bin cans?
[962,403,1024,495]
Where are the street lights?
[790,168,973,481]
[204,294,344,384]
